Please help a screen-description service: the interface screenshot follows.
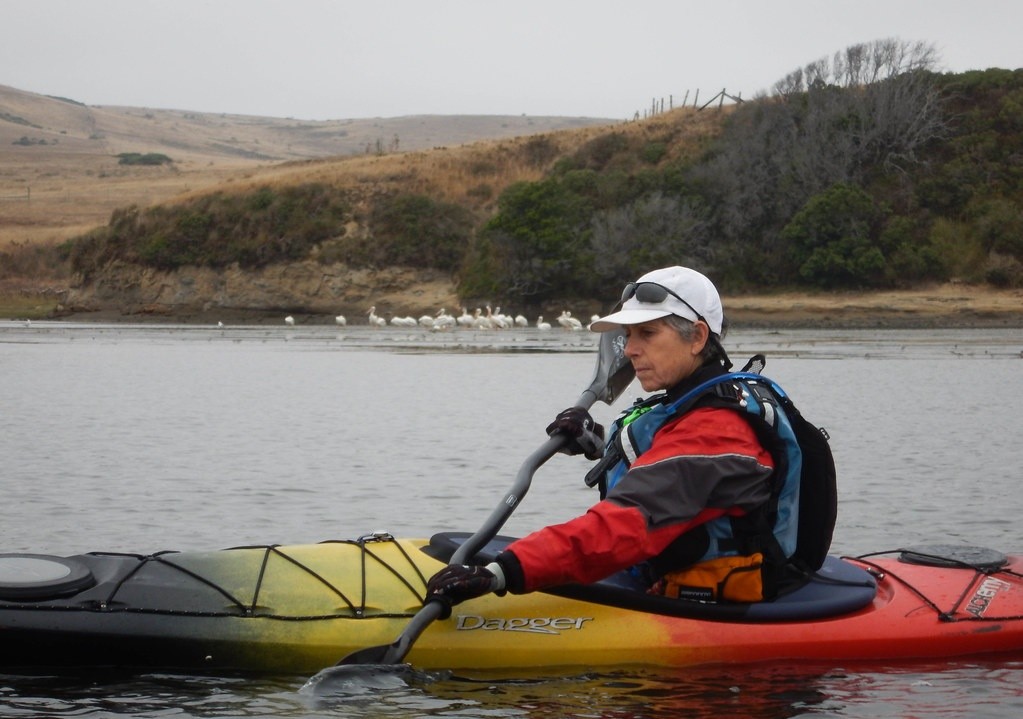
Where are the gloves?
[426,562,507,610]
[545,406,611,461]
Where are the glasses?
[621,282,711,334]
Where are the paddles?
[311,298,637,700]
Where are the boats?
[0,531,1023,668]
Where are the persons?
[424,266,778,605]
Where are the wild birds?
[364,306,387,329]
[515,315,528,328]
[390,306,514,329]
[536,316,551,331]
[335,315,346,329]
[283,315,295,326]
[216,321,224,329]
[555,310,582,328]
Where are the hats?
[589,265,723,336]
